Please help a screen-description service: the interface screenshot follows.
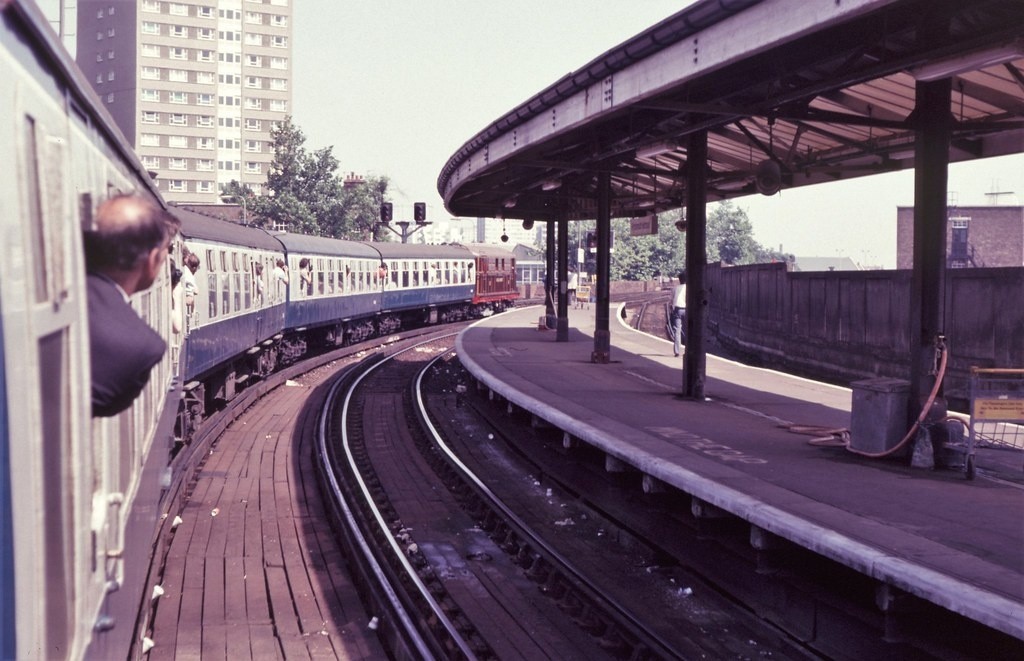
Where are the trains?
[0,1,520,661]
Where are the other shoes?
[675,354,679,357]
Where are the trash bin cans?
[850,376,912,463]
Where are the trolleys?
[575,285,591,310]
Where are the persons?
[256,263,263,293]
[277,260,289,284]
[300,258,311,284]
[671,272,687,357]
[468,263,473,268]
[82,194,169,418]
[432,263,436,268]
[379,263,387,277]
[453,262,458,271]
[170,258,184,309]
[568,265,577,305]
[181,246,200,305]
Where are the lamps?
[501,219,508,242]
[522,212,535,229]
[754,109,783,195]
[675,179,686,232]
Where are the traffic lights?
[381,204,390,220]
[415,205,423,221]
